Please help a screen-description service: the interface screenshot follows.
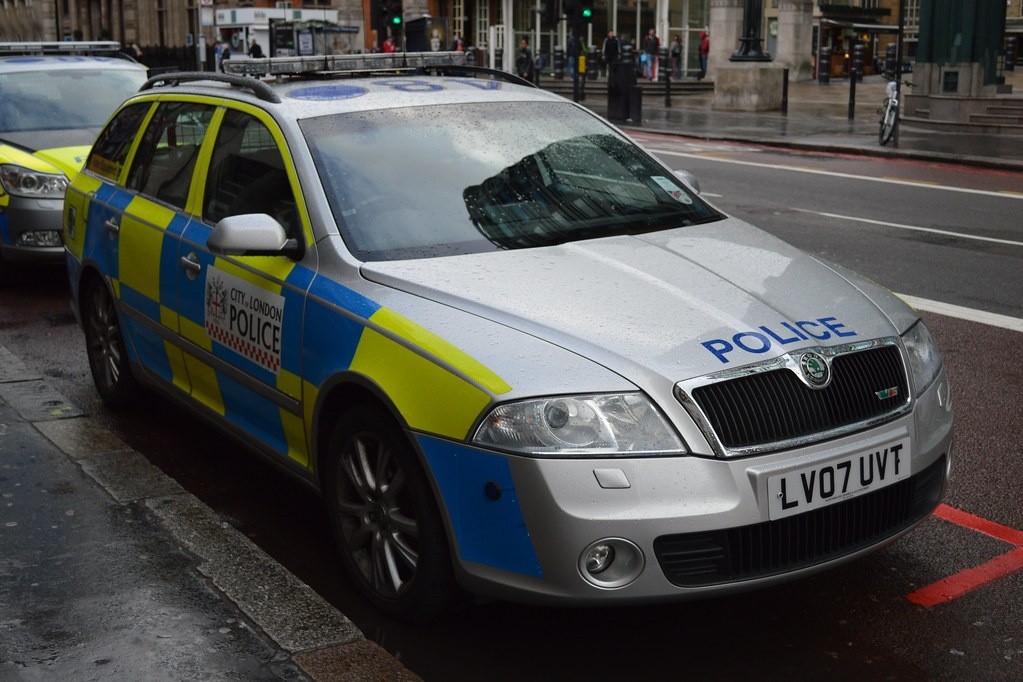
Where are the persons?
[601,28,620,79]
[64,8,479,98]
[698,31,710,74]
[669,34,682,80]
[565,29,584,81]
[515,37,534,84]
[643,28,660,80]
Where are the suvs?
[60,51,956,608]
[0,40,151,278]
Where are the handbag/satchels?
[640,51,648,65]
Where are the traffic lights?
[576,0,593,22]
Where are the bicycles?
[877,69,917,146]
